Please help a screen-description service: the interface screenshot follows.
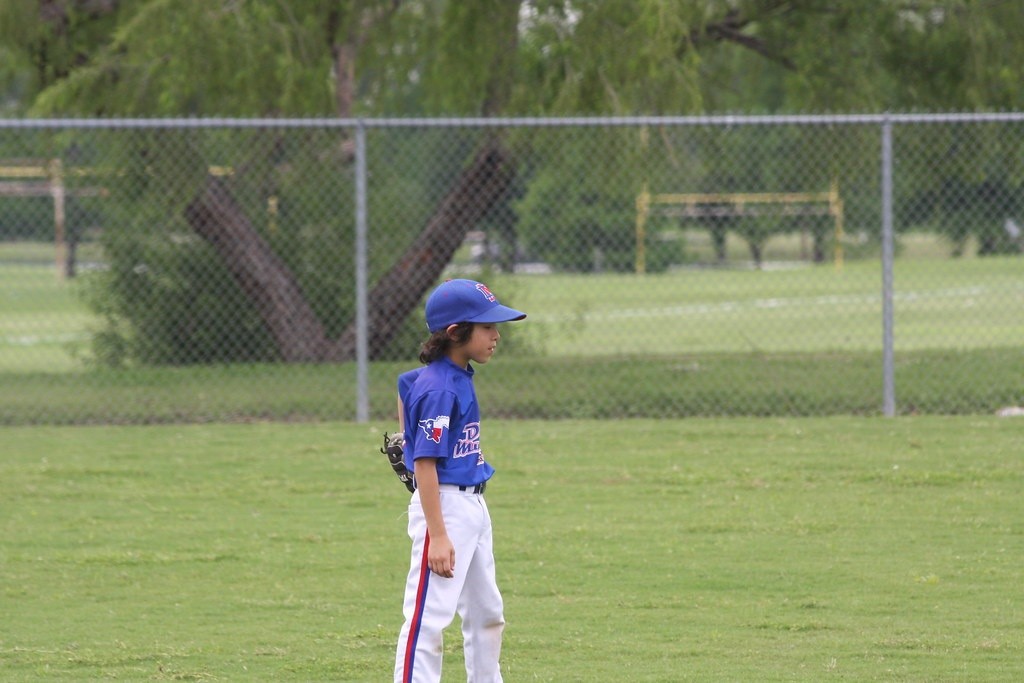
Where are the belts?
[459,481,486,495]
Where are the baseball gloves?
[385,433,417,493]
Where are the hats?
[424,279,526,332]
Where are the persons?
[393,279,527,683]
